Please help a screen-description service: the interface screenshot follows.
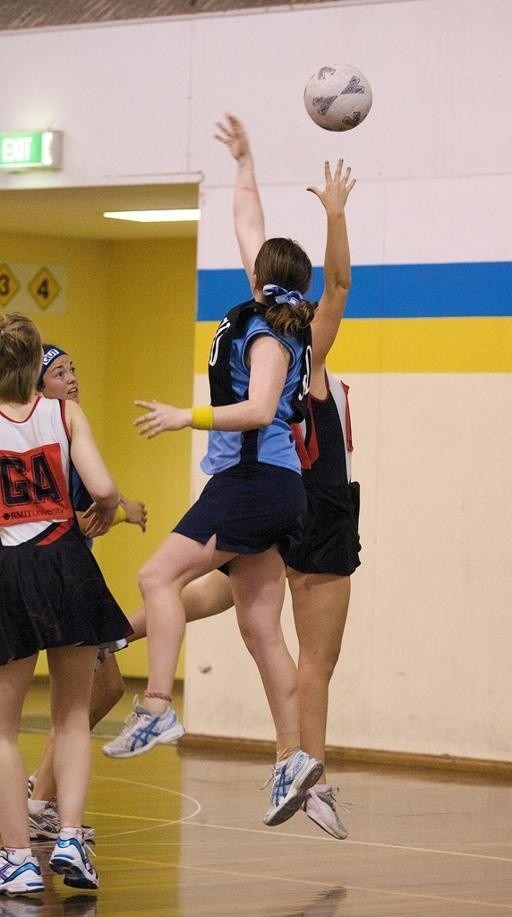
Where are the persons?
[99,156,360,842]
[3,309,121,899]
[31,337,146,840]
[106,110,323,830]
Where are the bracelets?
[191,401,215,432]
[110,503,128,528]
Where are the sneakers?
[263,750,349,840]
[101,705,184,759]
[0,776,99,893]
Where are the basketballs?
[305,63,373,131]
